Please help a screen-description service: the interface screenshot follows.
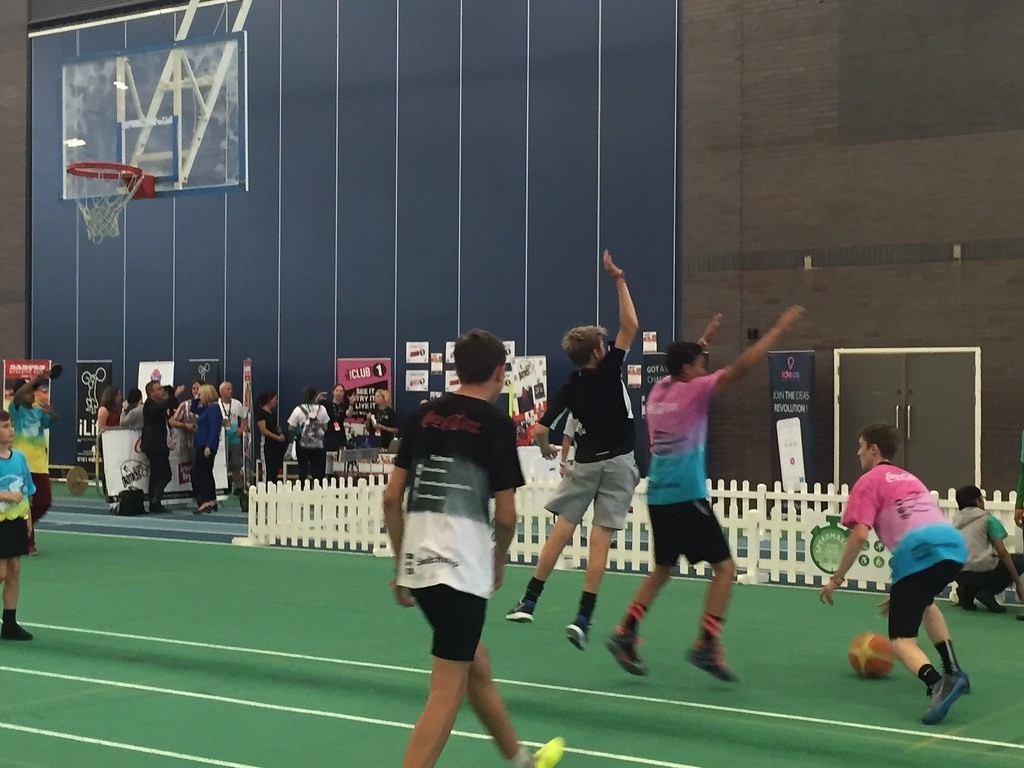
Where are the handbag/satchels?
[119,486,145,516]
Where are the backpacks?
[299,404,324,450]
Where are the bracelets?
[350,404,354,406]
[830,575,845,586]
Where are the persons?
[285,386,330,488]
[1013,431,1024,622]
[0,410,37,640]
[817,421,970,727]
[254,389,286,486]
[949,485,1024,614]
[6,369,60,556]
[505,249,640,650]
[365,389,399,450]
[383,328,564,768]
[98,380,249,514]
[606,305,803,680]
[316,384,356,482]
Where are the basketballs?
[848,635,896,677]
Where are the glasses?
[152,387,162,393]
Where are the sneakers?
[533,736,564,768]
[566,616,591,651]
[687,645,736,682]
[944,669,971,695]
[1,623,33,640]
[606,628,649,677]
[920,674,967,725]
[504,596,534,624]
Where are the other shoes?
[956,586,973,605]
[193,503,217,515]
[976,589,1006,613]
[150,504,171,513]
[27,543,37,556]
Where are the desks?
[332,461,397,485]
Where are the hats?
[13,378,40,394]
